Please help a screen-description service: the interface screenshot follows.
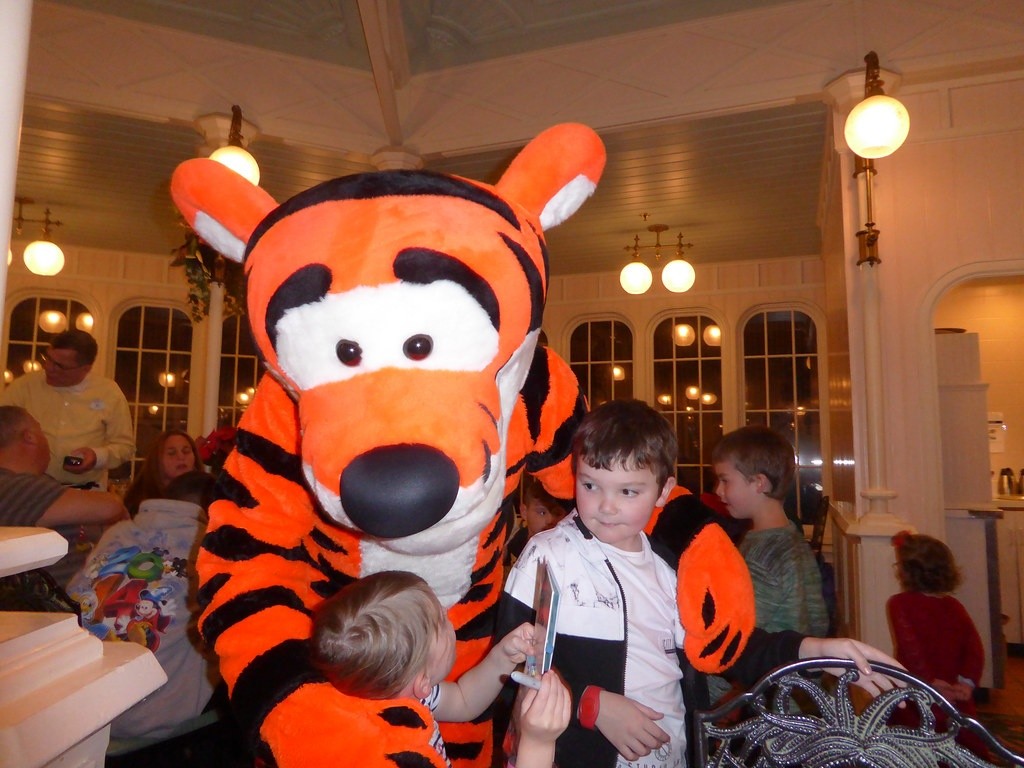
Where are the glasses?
[892,562,898,571]
[37,349,64,372]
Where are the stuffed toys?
[168,122,757,768]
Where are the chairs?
[686,655,1024,768]
[0,566,235,768]
[807,492,834,569]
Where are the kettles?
[997,467,1024,496]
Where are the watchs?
[577,685,607,731]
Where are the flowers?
[189,424,238,470]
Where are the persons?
[308,570,572,768]
[1,331,987,768]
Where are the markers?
[510,671,541,690]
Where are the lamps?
[4,196,68,279]
[619,222,697,294]
[842,49,912,272]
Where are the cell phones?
[535,563,560,677]
[63,456,83,465]
